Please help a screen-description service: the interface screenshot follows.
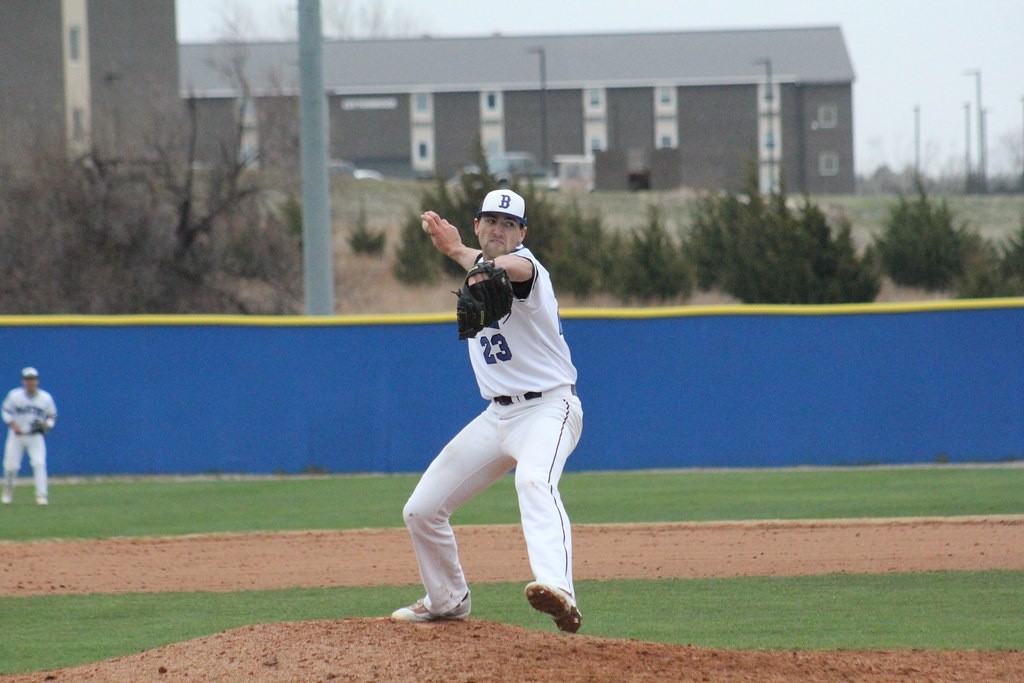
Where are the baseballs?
[421,219,434,234]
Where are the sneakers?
[526,582,583,633]
[390,590,472,621]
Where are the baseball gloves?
[457,262,513,340]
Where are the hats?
[475,188,527,224]
[22,368,39,378]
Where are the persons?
[0,367,59,506]
[390,189,583,634]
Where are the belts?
[495,385,578,407]
[14,428,43,435]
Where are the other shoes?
[37,495,49,507]
[1,484,12,504]
